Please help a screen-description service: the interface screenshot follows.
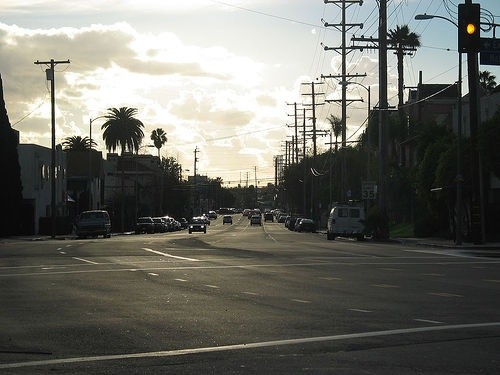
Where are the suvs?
[265,213,273,222]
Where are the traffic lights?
[457,3,481,54]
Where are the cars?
[188,217,207,233]
[135,216,188,234]
[208,207,262,226]
[273,210,316,233]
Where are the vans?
[79,211,111,240]
[326,206,365,243]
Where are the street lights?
[89,116,115,210]
[415,14,464,245]
[136,145,154,214]
[339,81,371,236]
